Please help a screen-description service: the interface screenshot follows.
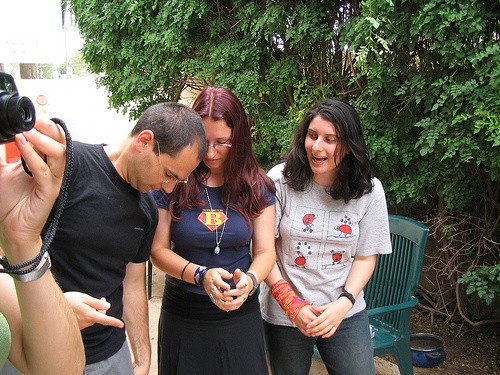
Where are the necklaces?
[201,176,231,255]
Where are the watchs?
[246,272,259,297]
[2,250,51,284]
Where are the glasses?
[207,140,232,151]
[152,139,189,185]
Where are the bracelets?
[181,262,191,283]
[338,289,357,306]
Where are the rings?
[211,286,217,293]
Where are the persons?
[250,98,393,375]
[40,100,209,375]
[0,117,87,375]
[149,86,277,375]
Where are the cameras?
[0,72,36,143]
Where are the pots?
[410,333,445,368]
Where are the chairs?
[314,215,429,375]
